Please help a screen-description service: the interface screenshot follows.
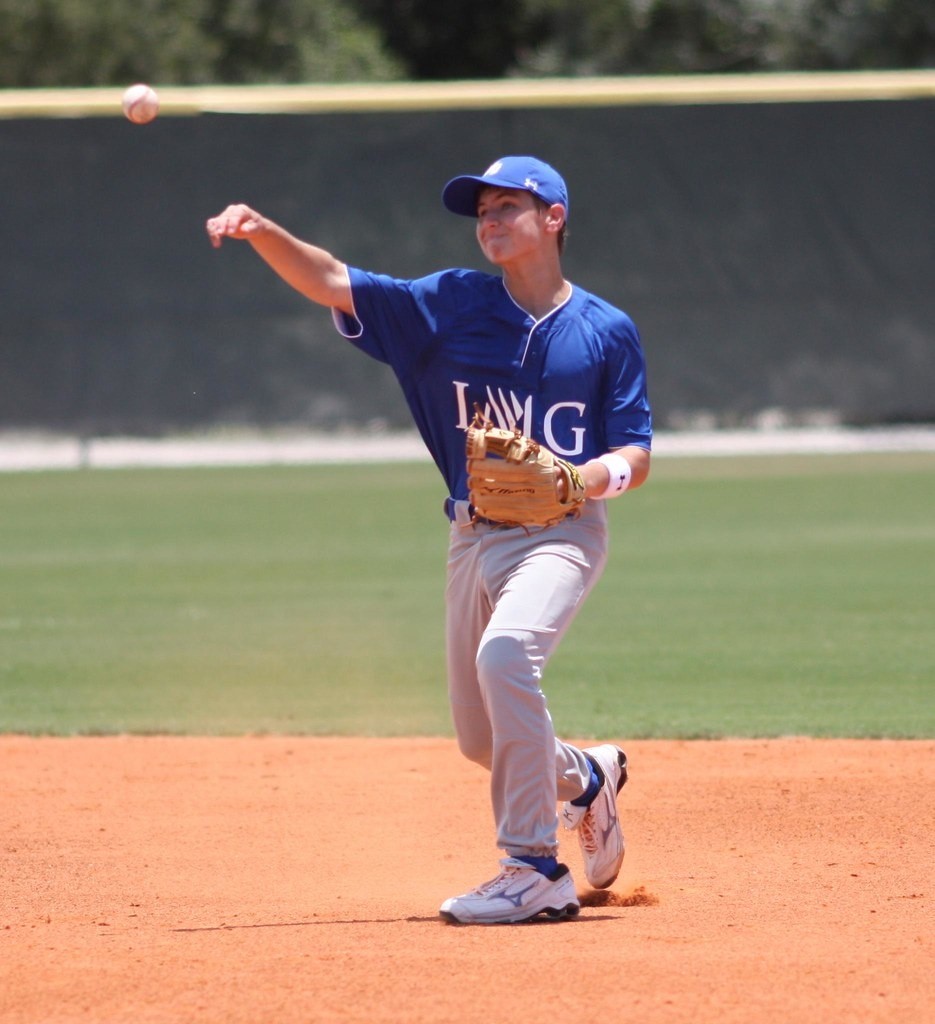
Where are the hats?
[442,156,568,219]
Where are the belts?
[447,497,499,526]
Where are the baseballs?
[124,84,161,124]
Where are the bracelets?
[587,453,632,502]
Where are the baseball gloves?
[465,419,589,529]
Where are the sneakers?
[439,858,580,925]
[561,744,628,889]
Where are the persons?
[206,153,653,928]
